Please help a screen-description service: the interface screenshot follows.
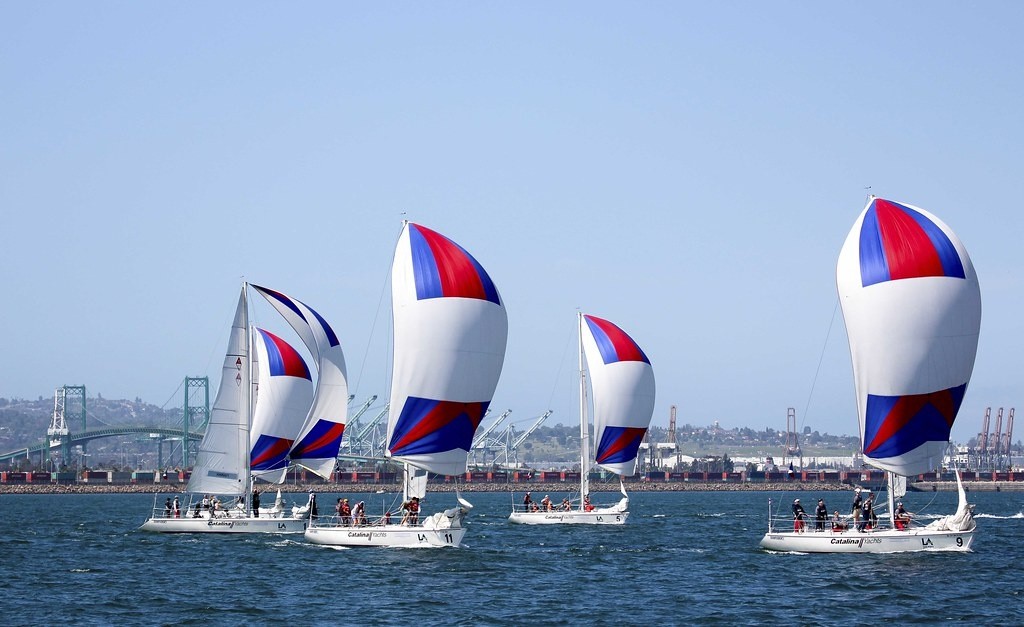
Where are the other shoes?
[858,528,861,532]
[861,531,866,533]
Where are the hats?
[794,498,800,502]
[855,488,861,493]
[898,502,903,506]
[344,499,349,502]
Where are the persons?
[336,498,350,527]
[850,488,875,533]
[203,495,209,508]
[831,510,845,529]
[194,500,201,518]
[209,495,228,518]
[792,499,809,533]
[253,491,260,517]
[165,497,172,518]
[524,492,532,512]
[531,501,540,512]
[400,497,421,527]
[584,495,589,505]
[351,501,366,527]
[816,498,828,529]
[172,496,180,518]
[541,494,553,512]
[562,499,571,511]
[894,502,908,531]
[381,513,390,523]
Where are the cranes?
[975,407,1017,471]
[664,404,677,447]
[777,407,805,461]
[331,394,395,464]
[463,407,557,472]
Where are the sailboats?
[509,311,657,526]
[136,278,348,534]
[304,217,510,551]
[760,188,983,555]
